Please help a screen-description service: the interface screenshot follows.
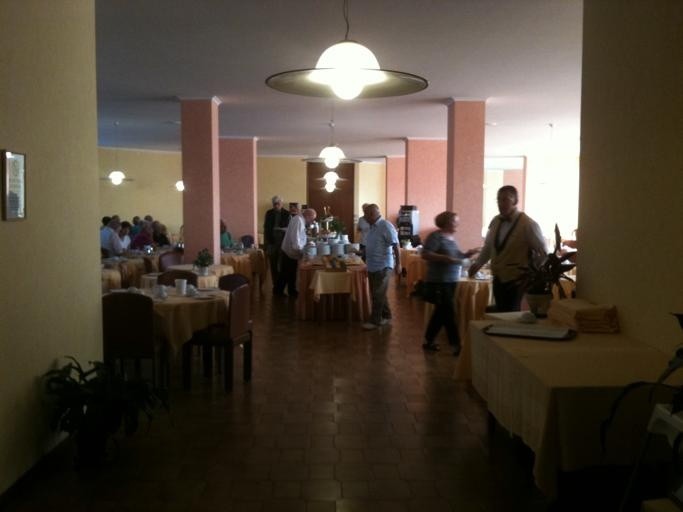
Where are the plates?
[341,234,349,245]
[307,247,317,257]
[317,245,330,256]
[482,325,577,341]
[328,239,339,245]
[332,244,345,256]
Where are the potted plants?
[506,223,578,318]
[39,355,113,480]
[192,248,214,276]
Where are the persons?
[264,196,291,296]
[350,204,402,329]
[176,225,184,249]
[112,215,121,224]
[273,209,316,296]
[220,219,237,248]
[415,211,480,355]
[129,216,172,251]
[102,216,111,226]
[108,222,131,259]
[469,186,548,312]
[101,219,134,259]
[288,203,300,217]
[357,203,370,262]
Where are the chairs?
[158,269,198,290]
[180,285,252,393]
[215,274,252,383]
[102,292,157,392]
[532,382,683,512]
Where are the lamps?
[301,103,363,170]
[266,1,427,100]
[99,121,133,185]
[173,121,185,191]
[315,143,349,192]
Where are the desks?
[404,254,432,297]
[458,318,683,455]
[399,248,419,276]
[314,270,357,328]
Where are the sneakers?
[362,322,378,330]
[380,318,391,325]
[422,341,441,352]
[454,344,463,355]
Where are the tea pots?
[152,283,172,299]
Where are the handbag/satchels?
[422,231,445,305]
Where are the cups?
[175,279,187,297]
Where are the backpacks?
[417,280,443,305]
